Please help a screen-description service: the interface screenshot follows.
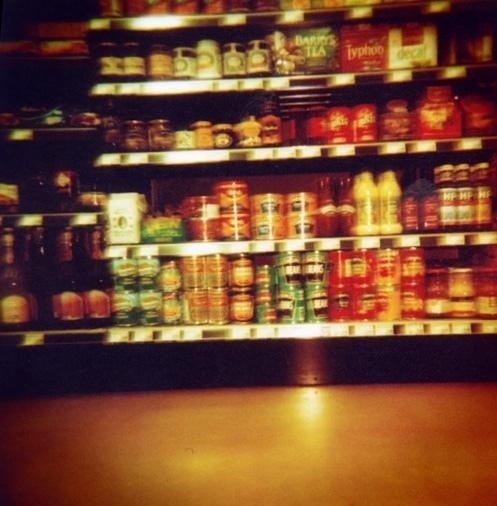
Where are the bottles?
[0,222,112,328]
[316,160,491,235]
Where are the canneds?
[101,80,497,153]
[110,245,497,328]
[94,0,497,81]
[1,1,112,328]
[104,161,497,247]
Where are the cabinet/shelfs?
[0,0,496,348]
